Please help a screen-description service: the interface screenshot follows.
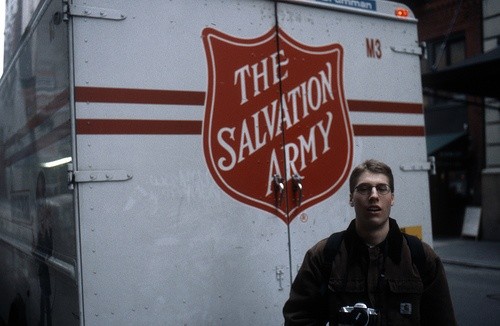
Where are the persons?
[282,159,457,326]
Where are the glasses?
[352,183,392,195]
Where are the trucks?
[0,0,432,326]
